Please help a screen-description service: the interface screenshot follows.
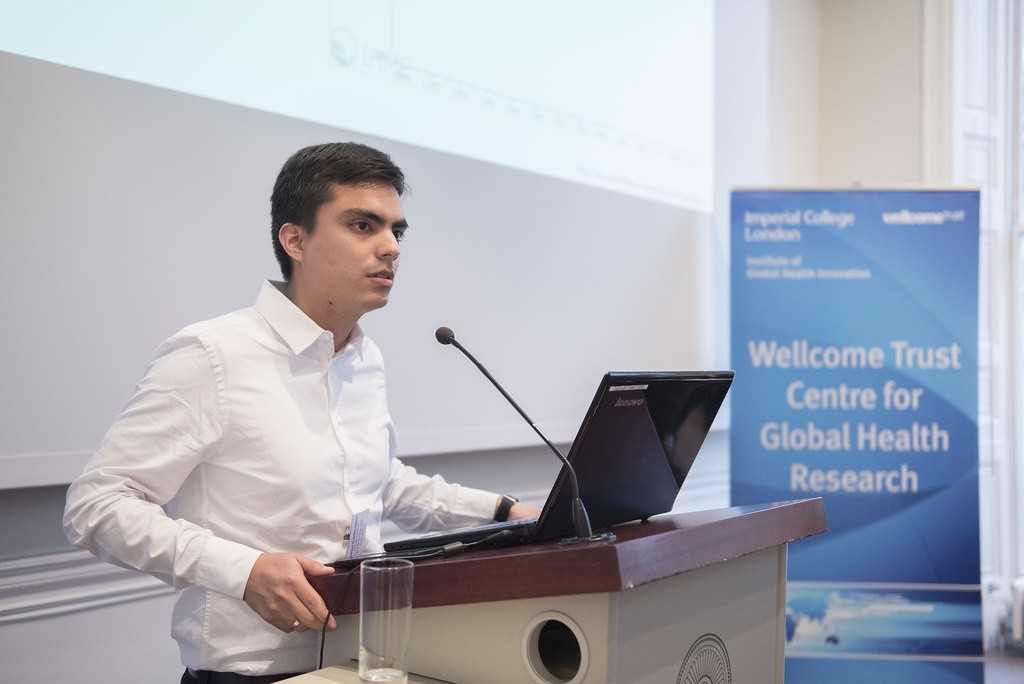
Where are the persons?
[62,142,544,684]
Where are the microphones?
[435,327,592,540]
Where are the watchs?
[492,495,520,524]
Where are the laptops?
[383,370,737,552]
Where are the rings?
[288,618,300,628]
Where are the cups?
[358,557,414,684]
